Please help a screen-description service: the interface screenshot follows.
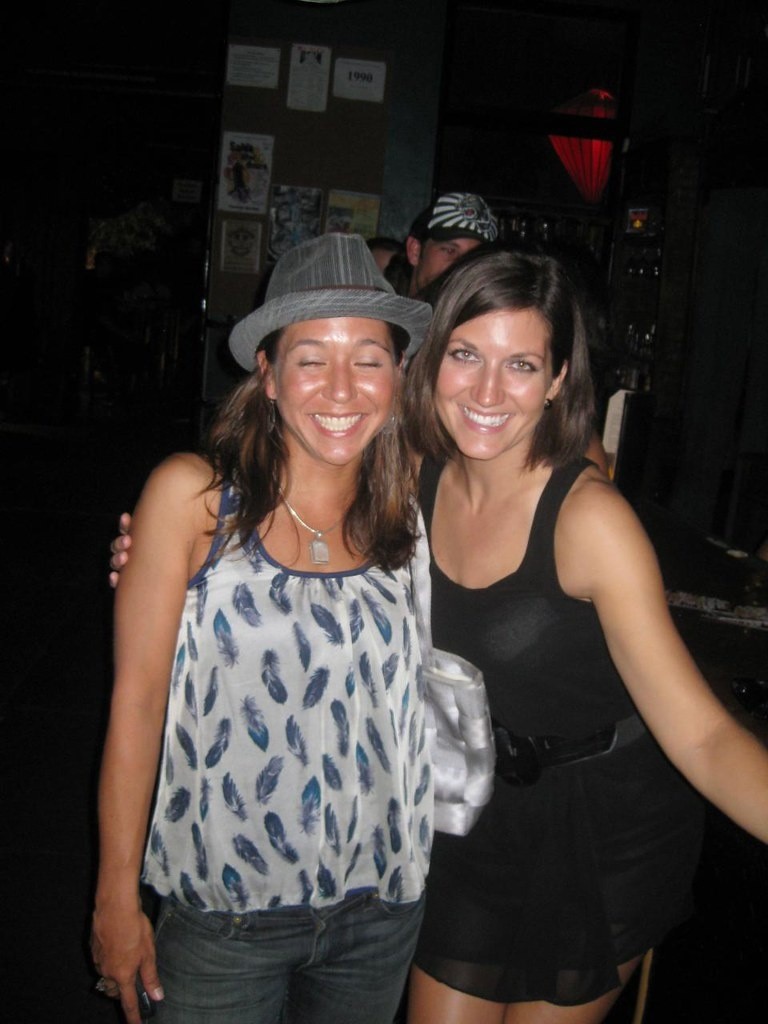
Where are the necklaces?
[278,481,358,568]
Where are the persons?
[90,231,436,1024]
[107,246,768,1024]
[367,183,496,357]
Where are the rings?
[96,977,106,992]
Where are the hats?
[409,189,502,246]
[228,232,433,372]
